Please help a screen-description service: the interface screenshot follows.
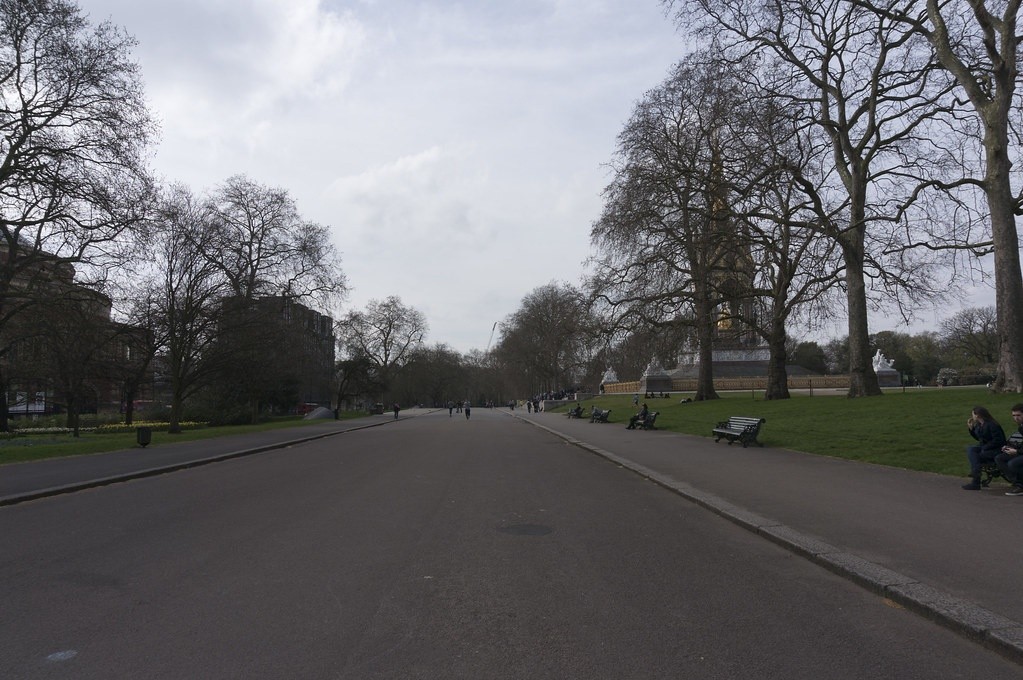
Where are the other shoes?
[1005,488,1023,496]
[632,426,635,429]
[962,482,980,490]
[626,427,631,429]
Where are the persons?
[912,373,948,391]
[488,398,540,414]
[994,403,1023,496]
[600,383,605,394]
[633,390,693,406]
[393,400,400,419]
[566,403,648,430]
[962,406,1006,490]
[448,398,472,421]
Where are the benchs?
[594,409,611,424]
[979,432,1023,486]
[635,411,660,430]
[712,416,766,448]
[568,408,585,418]
